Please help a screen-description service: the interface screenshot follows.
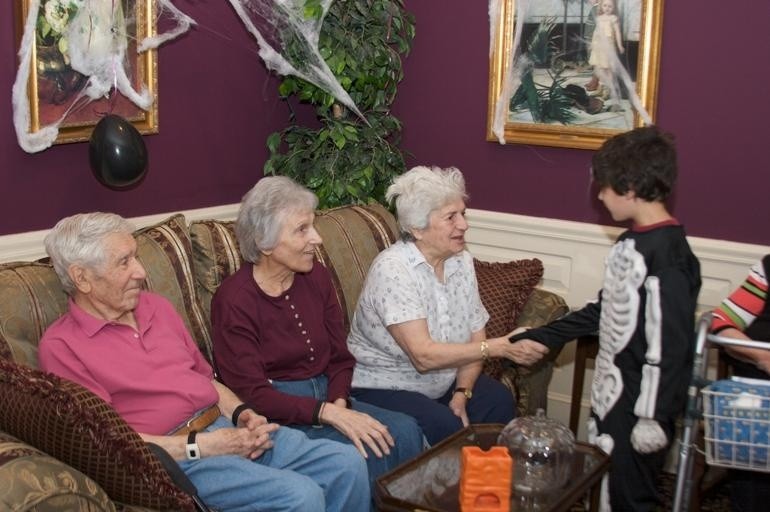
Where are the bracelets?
[232,402,250,426]
[480,340,489,361]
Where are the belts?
[169,405,220,436]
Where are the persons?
[39,211,371,512]
[347,165,516,448]
[509,128,701,512]
[583,0,605,90]
[696,255,770,511]
[211,176,423,499]
[589,0,626,101]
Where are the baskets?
[699,378,770,473]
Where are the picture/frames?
[486,1,665,150]
[14,0,160,145]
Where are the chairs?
[688,341,729,511]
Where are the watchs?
[186,431,201,461]
[453,388,473,400]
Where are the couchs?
[0,203,569,512]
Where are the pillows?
[0,359,195,512]
[470,257,544,377]
